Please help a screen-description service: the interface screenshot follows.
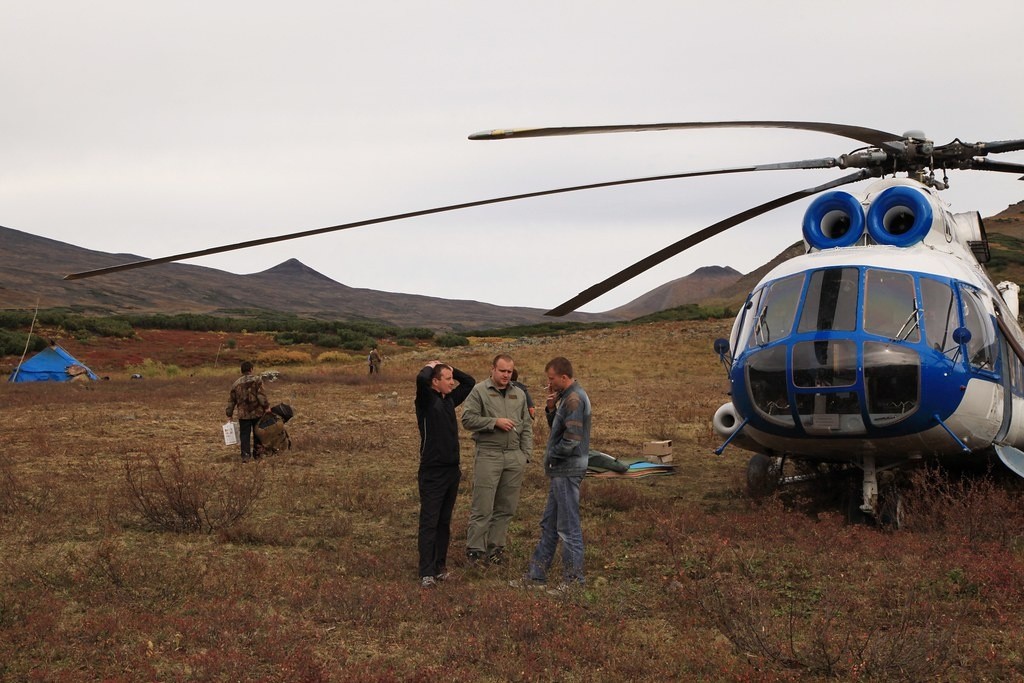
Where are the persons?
[510,369,535,415]
[508,357,592,595]
[367,347,381,374]
[461,354,534,572]
[414,360,476,589]
[225,361,272,464]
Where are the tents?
[8,340,100,381]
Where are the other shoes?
[508,574,546,592]
[544,582,579,599]
[420,575,437,593]
[462,557,488,575]
[434,572,450,582]
[482,555,504,567]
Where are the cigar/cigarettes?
[513,426,516,430]
[544,384,550,390]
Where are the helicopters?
[63,122,1023,525]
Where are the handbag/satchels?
[264,402,293,424]
[254,411,292,455]
[222,419,241,446]
